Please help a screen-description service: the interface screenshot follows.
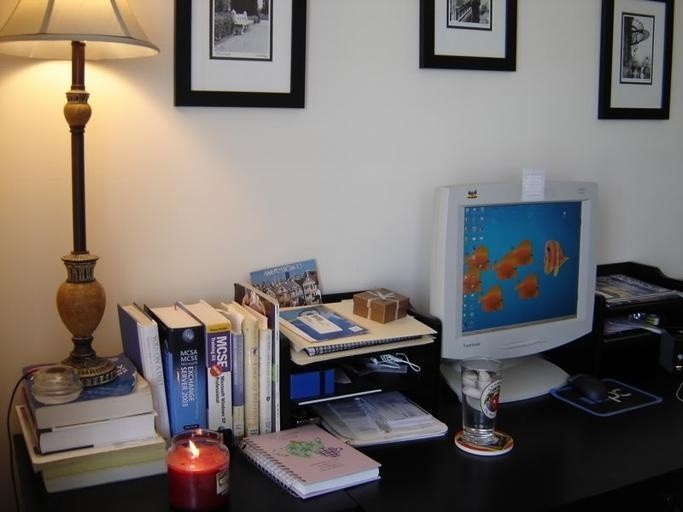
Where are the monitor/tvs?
[429,180,599,403]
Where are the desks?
[11,335,683,512]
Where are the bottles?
[165,429,230,511]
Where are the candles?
[167,441,231,512]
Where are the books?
[13,281,450,500]
[594,273,679,307]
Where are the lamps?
[0,0,158,383]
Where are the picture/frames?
[597,0,674,120]
[418,0,518,72]
[173,1,308,108]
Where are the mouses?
[571,376,609,401]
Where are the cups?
[461,359,503,445]
